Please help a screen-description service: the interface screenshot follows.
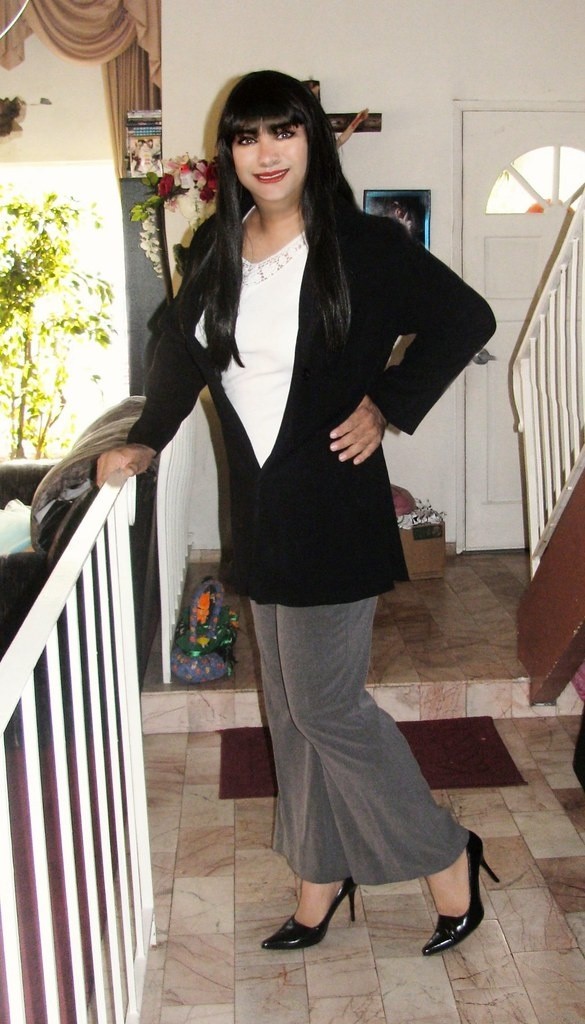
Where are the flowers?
[134,154,217,284]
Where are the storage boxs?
[397,505,446,582]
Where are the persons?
[96,70,500,954]
[392,201,412,235]
[126,138,154,172]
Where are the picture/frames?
[363,189,432,250]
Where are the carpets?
[216,717,528,801]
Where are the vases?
[200,393,236,592]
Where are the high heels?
[420,829,500,956]
[261,877,358,950]
